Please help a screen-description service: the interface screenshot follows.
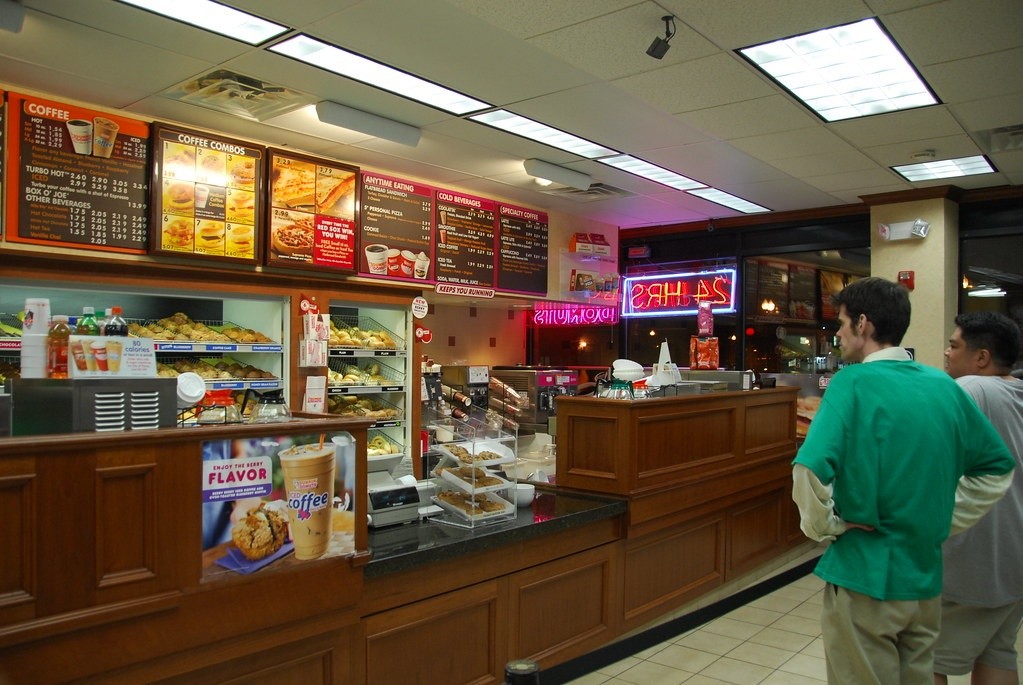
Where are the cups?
[278,443,336,561]
[453,392,471,406]
[451,407,469,421]
[20,298,51,378]
[365,244,388,275]
[176,372,206,407]
[436,425,454,441]
[71,341,122,371]
[414,254,430,279]
[195,187,209,208]
[66,120,93,155]
[93,117,119,158]
[400,250,416,277]
[387,249,401,276]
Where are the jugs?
[593,378,650,401]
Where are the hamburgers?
[199,221,225,247]
[230,227,254,251]
[227,192,254,218]
[167,183,195,211]
[164,219,194,247]
[231,162,256,184]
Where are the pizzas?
[273,223,315,258]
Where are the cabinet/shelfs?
[329,314,409,483]
[1,311,285,427]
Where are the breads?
[326,321,400,456]
[128,312,272,342]
[436,444,506,517]
[174,393,258,421]
[154,359,273,379]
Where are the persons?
[791,276,1018,685]
[933,309,1023,685]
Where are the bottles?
[99,308,113,336]
[48,315,73,378]
[596,272,619,294]
[421,425,428,452]
[105,307,128,337]
[77,306,100,336]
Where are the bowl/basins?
[508,484,535,507]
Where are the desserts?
[232,499,289,560]
